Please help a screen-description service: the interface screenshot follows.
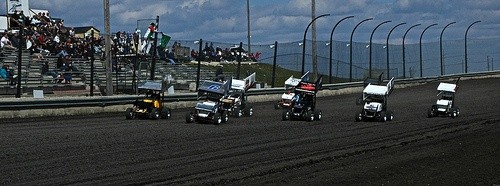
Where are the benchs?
[0,47,237,88]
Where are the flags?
[161,34,171,49]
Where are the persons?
[0,10,105,81]
[191,46,236,62]
[113,23,177,58]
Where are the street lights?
[193,39,202,92]
[324,15,482,84]
[269,40,278,88]
[299,14,329,78]
